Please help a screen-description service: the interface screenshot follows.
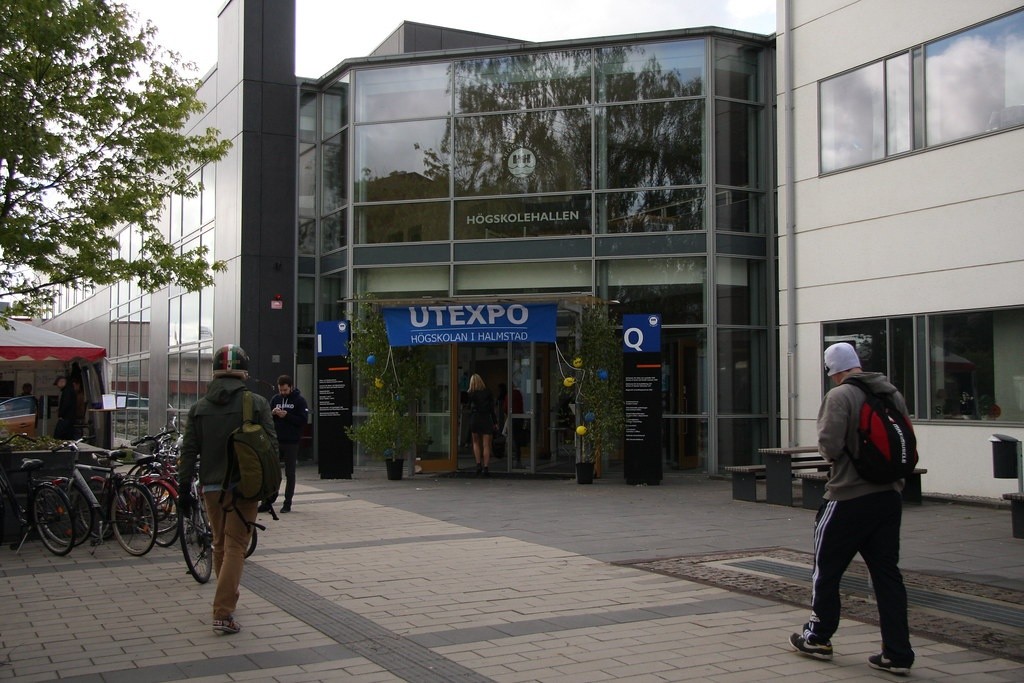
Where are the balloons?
[576,426,588,435]
[585,413,595,422]
[367,355,376,366]
[374,378,385,388]
[597,368,608,380]
[384,449,393,457]
[572,357,584,368]
[563,378,574,386]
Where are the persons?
[177,343,282,632]
[504,378,524,464]
[53,375,86,440]
[467,373,499,472]
[12,383,38,428]
[258,375,308,512]
[789,341,914,672]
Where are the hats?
[52,376,65,385]
[823,342,860,376]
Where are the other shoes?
[481,467,489,479]
[257,504,270,512]
[476,463,483,476]
[280,506,291,513]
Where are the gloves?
[178,495,199,518]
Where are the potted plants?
[345,291,432,480]
[553,299,627,485]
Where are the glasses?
[824,366,831,374]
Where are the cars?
[110,389,175,420]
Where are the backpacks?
[67,389,86,421]
[217,391,282,512]
[834,377,920,485]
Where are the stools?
[1003,493,1024,539]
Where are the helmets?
[212,344,250,379]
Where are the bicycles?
[1,415,259,585]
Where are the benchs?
[795,468,927,510]
[757,446,818,506]
[725,462,833,502]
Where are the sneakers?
[788,632,834,660]
[212,615,241,634]
[866,652,912,675]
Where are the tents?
[0,314,108,394]
